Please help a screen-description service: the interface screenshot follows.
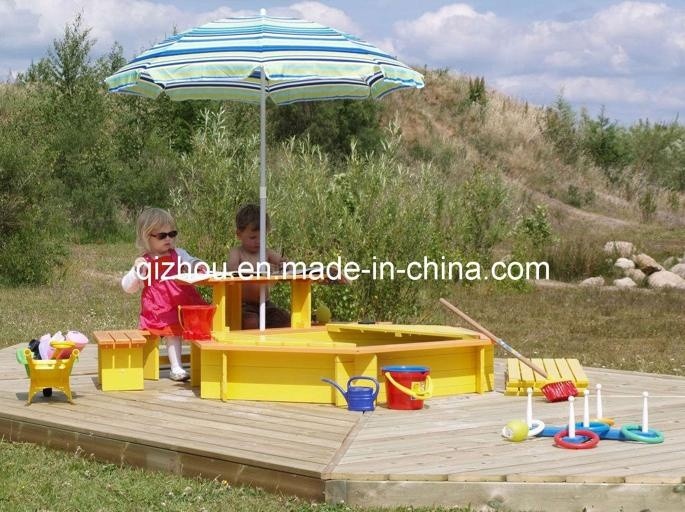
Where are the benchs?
[92,328,161,391]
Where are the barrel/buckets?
[381,365,431,410]
[176,304,217,341]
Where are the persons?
[121,207,210,381]
[225,203,291,329]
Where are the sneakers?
[168,370,190,382]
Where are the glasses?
[149,230,179,240]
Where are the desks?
[174,273,328,330]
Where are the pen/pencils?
[281,247,285,257]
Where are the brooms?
[438,298,579,402]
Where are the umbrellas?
[102,8,425,330]
